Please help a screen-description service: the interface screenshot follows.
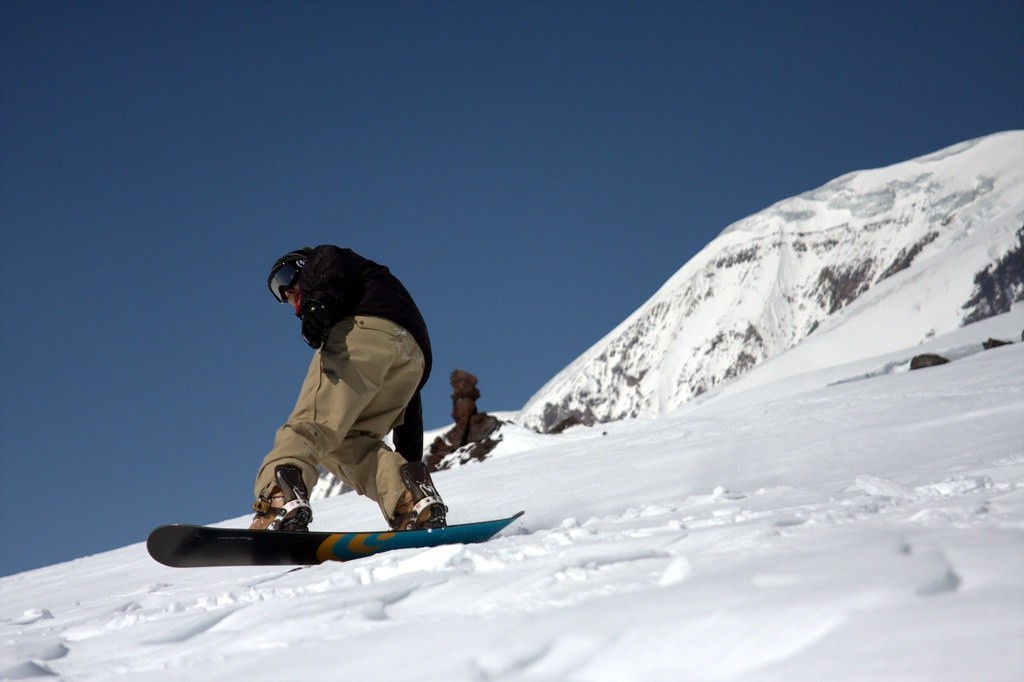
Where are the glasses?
[268,258,307,303]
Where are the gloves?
[301,300,331,349]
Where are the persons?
[248,245,446,532]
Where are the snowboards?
[146,511,527,568]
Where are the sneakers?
[389,489,444,531]
[249,487,312,529]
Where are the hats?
[271,247,313,272]
